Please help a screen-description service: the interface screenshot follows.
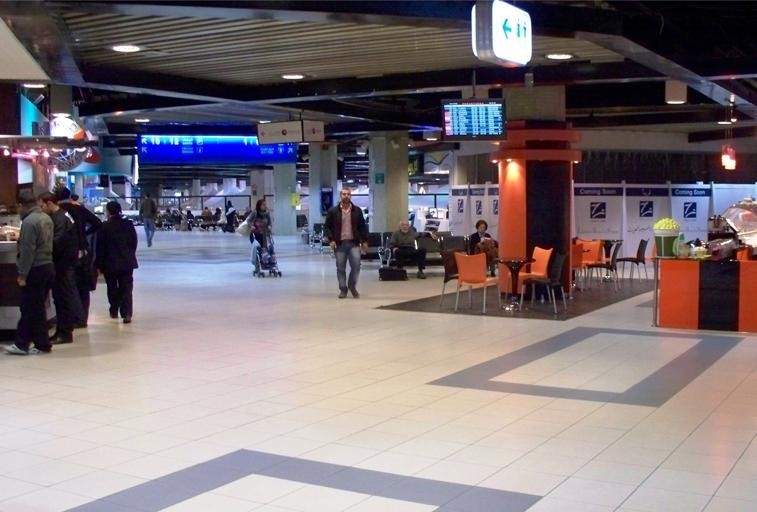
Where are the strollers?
[250,229,281,278]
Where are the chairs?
[309,220,446,275]
[437,231,651,316]
[129,210,241,231]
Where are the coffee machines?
[707,214,741,254]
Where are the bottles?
[677,232,685,257]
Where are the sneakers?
[338,286,359,298]
[2,342,28,354]
[417,271,425,279]
[30,347,51,355]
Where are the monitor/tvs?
[440,97,507,141]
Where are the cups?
[680,245,711,260]
[653,228,678,257]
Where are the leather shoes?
[47,333,74,344]
[109,309,131,323]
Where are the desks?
[729,251,756,334]
[652,250,718,331]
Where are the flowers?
[655,214,680,229]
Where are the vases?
[654,232,681,260]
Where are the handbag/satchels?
[234,217,252,237]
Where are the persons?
[389,219,438,279]
[246,199,272,243]
[363,209,369,232]
[260,245,271,265]
[469,220,498,277]
[324,188,370,298]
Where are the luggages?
[379,267,408,281]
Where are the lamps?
[721,142,739,175]
[662,74,690,109]
[712,95,737,128]
[355,145,368,157]
